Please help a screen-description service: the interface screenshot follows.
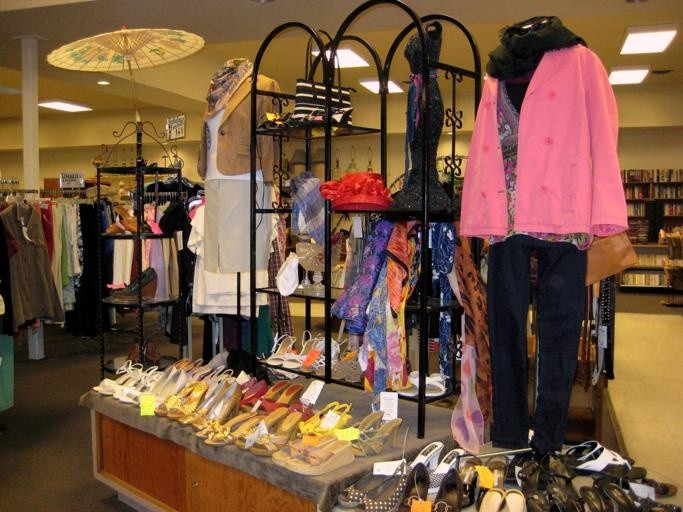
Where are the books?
[620,169,683,285]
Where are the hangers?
[0,174,190,209]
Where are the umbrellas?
[46,25,205,122]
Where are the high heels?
[111,267,158,297]
[93,358,405,475]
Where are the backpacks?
[293,78,353,126]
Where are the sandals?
[265,331,447,397]
[105,205,143,234]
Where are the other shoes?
[127,338,142,366]
[339,441,679,509]
[144,338,159,367]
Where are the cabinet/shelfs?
[249,1,480,438]
[92,122,189,380]
[617,182,683,291]
[81,362,460,512]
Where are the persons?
[198,58,282,274]
[460,16,629,451]
